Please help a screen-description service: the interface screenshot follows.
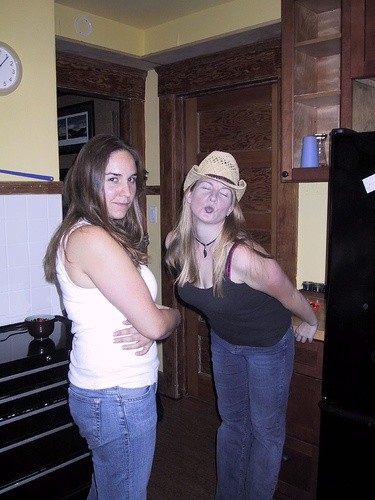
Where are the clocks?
[0,40,25,99]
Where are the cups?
[306,297,320,312]
[300,136,319,168]
[302,281,325,293]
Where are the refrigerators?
[315,128,375,500]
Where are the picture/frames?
[57,99,104,158]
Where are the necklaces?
[192,228,223,258]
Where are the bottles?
[314,134,328,168]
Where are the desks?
[0,315,108,500]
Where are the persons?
[164,150,317,500]
[43,132,181,500]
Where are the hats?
[183,151,247,202]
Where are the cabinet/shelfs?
[277,1,375,190]
[268,284,326,467]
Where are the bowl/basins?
[24,314,55,340]
